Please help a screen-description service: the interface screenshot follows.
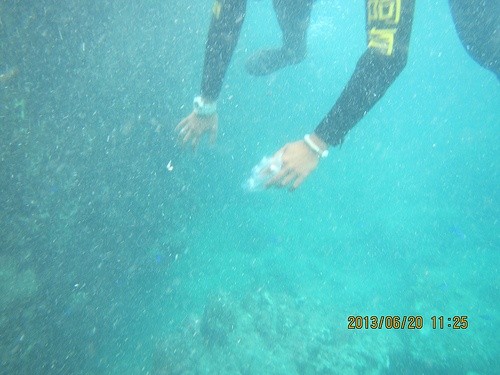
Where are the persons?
[172,0,499,195]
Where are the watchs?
[192,96,219,117]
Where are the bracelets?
[302,135,329,158]
